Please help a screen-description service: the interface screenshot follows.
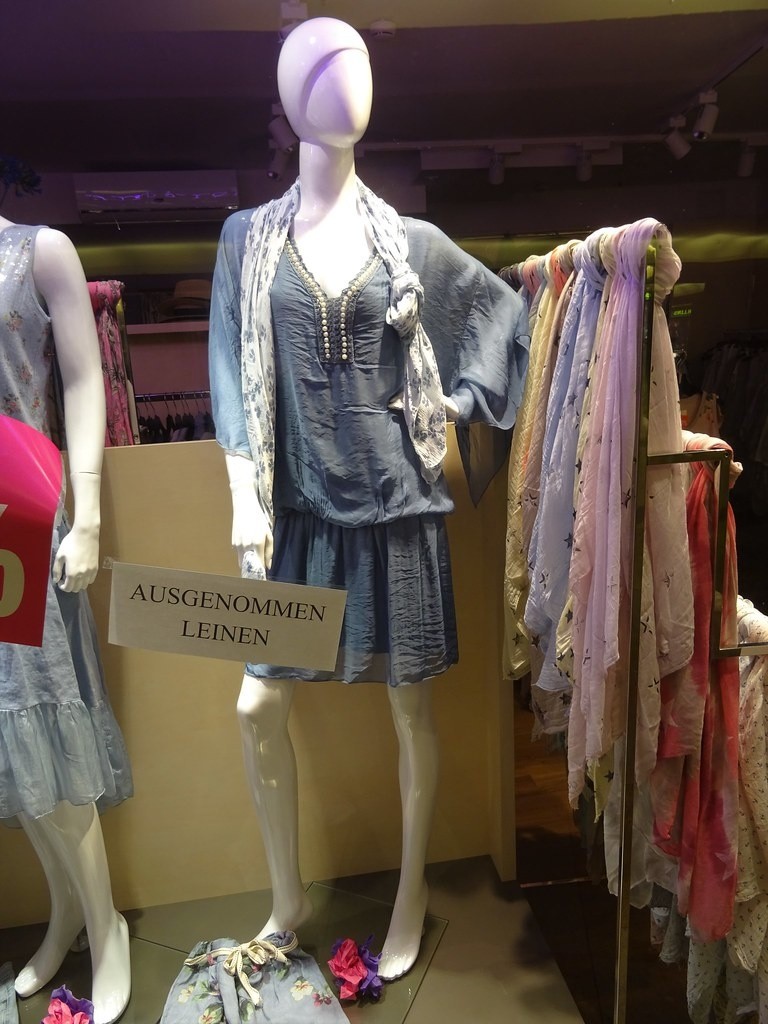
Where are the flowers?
[0,154,43,212]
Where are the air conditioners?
[74,169,240,223]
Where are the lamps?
[573,142,591,181]
[487,152,503,186]
[737,137,754,178]
[663,115,691,161]
[692,91,719,140]
[268,114,299,180]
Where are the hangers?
[133,390,215,436]
[701,328,768,363]
[676,348,722,407]
